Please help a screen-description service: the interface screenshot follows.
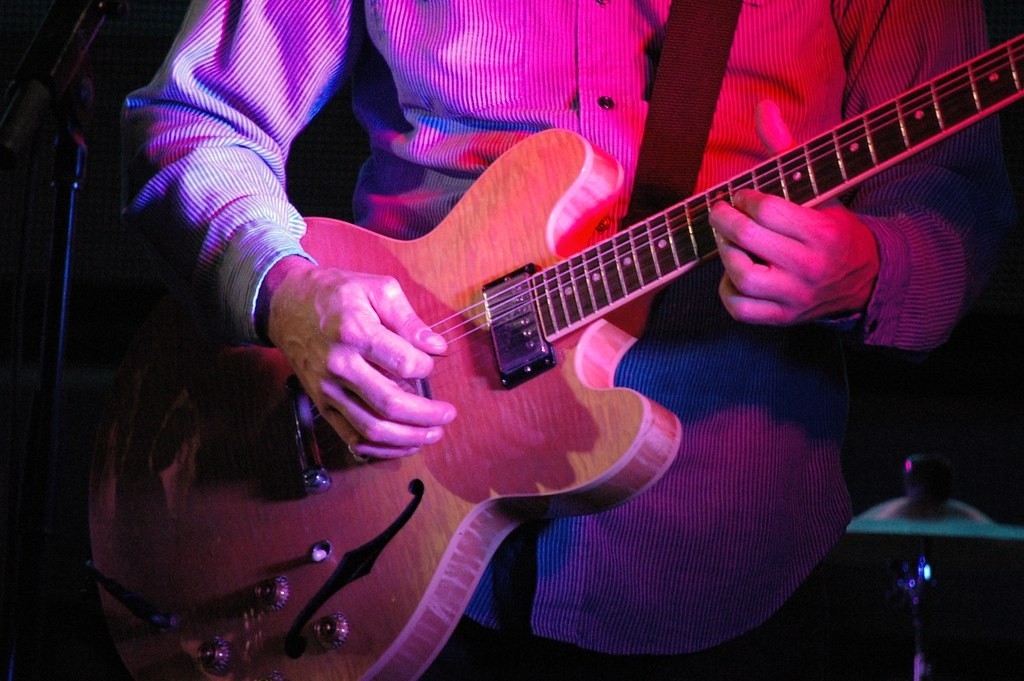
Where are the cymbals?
[846,496,1024,540]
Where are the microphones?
[0,0,120,171]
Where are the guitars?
[77,33,1024,681]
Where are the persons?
[123,0,1007,681]
